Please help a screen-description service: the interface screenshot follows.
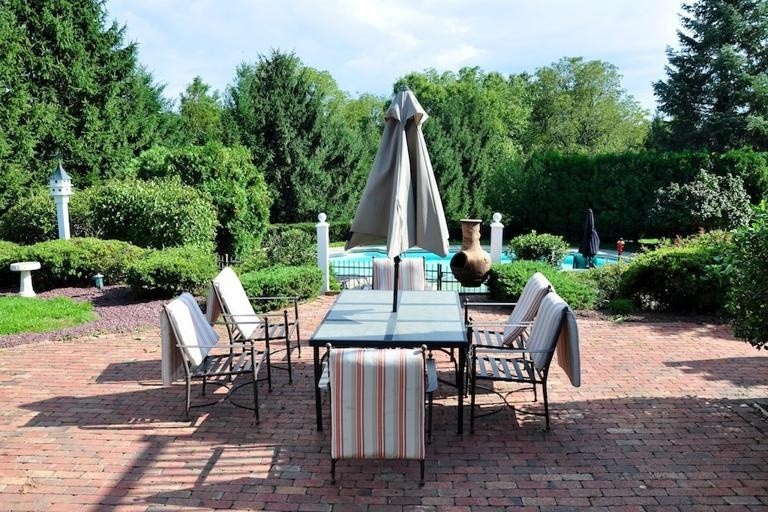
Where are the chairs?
[324,343,435,487]
[463,285,552,369]
[371,255,426,288]
[162,302,273,426]
[462,289,568,434]
[210,275,301,384]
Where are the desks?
[308,289,468,435]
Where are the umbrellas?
[343,83,450,311]
[578,208,600,269]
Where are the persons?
[617,237,625,255]
[640,244,645,252]
